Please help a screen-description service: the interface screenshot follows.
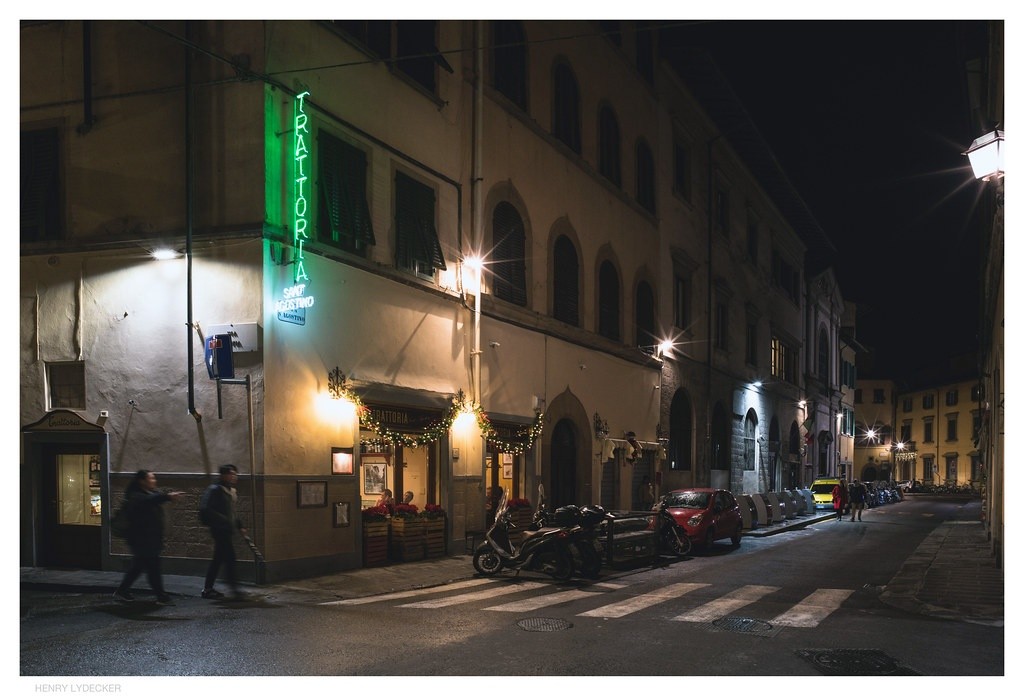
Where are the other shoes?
[115,589,135,602]
[837,516,842,521]
[157,594,177,606]
[858,516,862,522]
[850,518,854,521]
[201,589,223,598]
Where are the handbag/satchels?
[111,510,128,538]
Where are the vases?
[391,516,425,563]
[509,507,533,545]
[361,517,389,567]
[418,516,446,560]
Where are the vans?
[810,478,848,510]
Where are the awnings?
[920,453,934,458]
[944,452,958,458]
[967,451,980,457]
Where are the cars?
[846,481,902,508]
[643,488,744,553]
[897,479,921,493]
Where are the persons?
[849,479,867,521]
[486,487,503,515]
[111,469,184,606]
[404,491,414,505]
[380,489,394,504]
[832,480,849,522]
[199,463,249,600]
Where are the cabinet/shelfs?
[89,487,101,516]
[594,509,662,571]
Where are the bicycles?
[917,481,977,495]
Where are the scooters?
[471,486,583,581]
[529,484,607,578]
[613,490,692,558]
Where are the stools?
[465,530,488,555]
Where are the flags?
[804,412,816,430]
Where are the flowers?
[360,502,445,524]
[507,497,531,512]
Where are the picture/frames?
[296,479,328,509]
[502,452,513,465]
[333,502,351,528]
[503,464,512,479]
[363,463,387,495]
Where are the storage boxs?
[555,504,605,528]
